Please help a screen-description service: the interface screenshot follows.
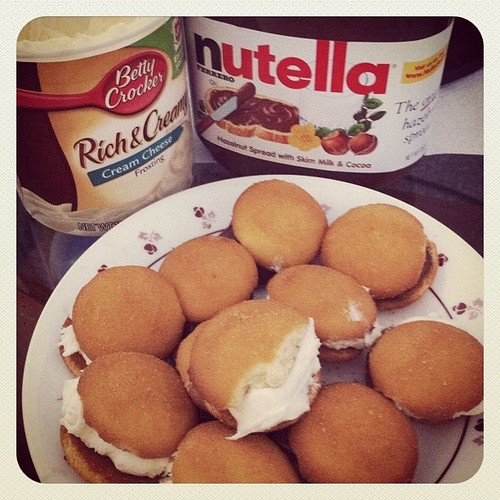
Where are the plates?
[20,174,483,483]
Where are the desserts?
[57,180,484,484]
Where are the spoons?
[16,50,168,115]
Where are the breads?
[206,85,300,144]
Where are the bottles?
[17,17,469,304]
